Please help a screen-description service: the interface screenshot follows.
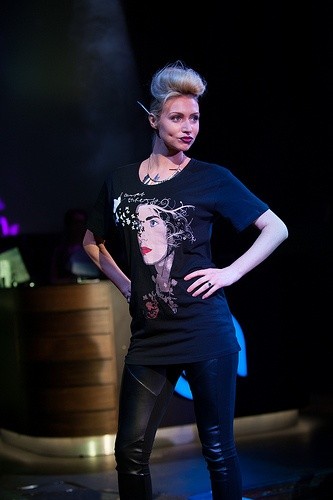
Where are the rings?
[207,282,212,287]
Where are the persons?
[79,66,291,499]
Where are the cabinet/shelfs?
[14,279,132,437]
